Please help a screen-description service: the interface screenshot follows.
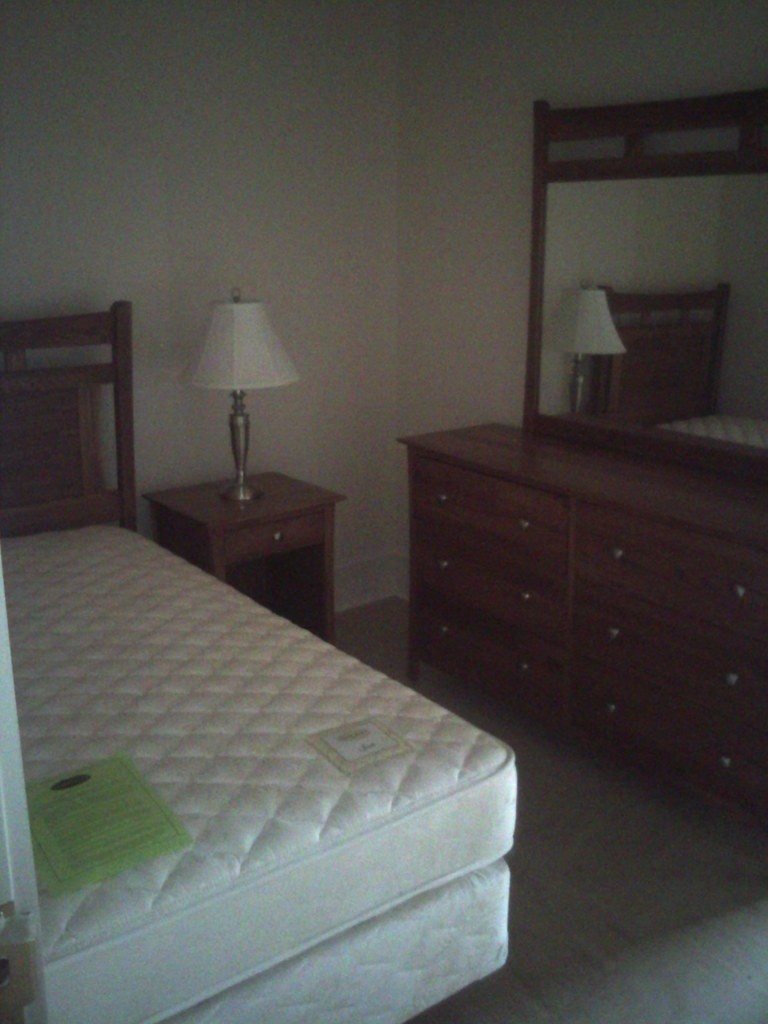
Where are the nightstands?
[141,471,346,647]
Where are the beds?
[595,282,767,451]
[1,299,516,1024]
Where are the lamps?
[547,288,626,414]
[186,300,301,504]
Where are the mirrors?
[519,99,768,480]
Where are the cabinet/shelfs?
[395,423,767,826]
